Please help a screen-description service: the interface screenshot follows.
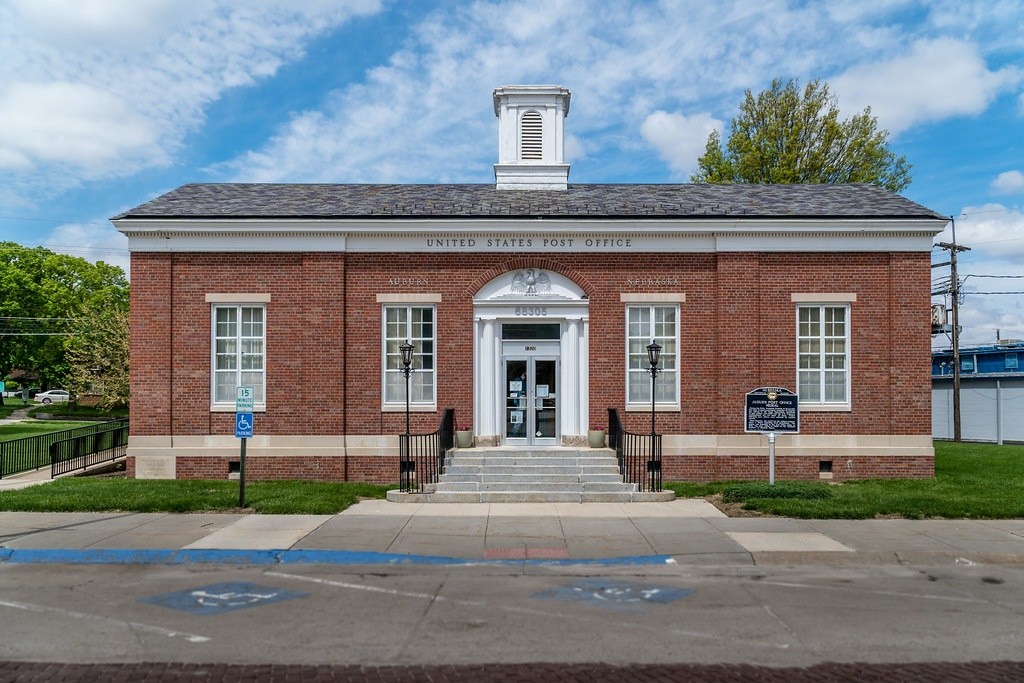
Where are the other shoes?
[536,431,542,436]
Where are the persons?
[508,368,543,437]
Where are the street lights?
[398,338,416,493]
[643,341,665,492]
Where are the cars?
[13,387,43,399]
[34,389,68,405]
